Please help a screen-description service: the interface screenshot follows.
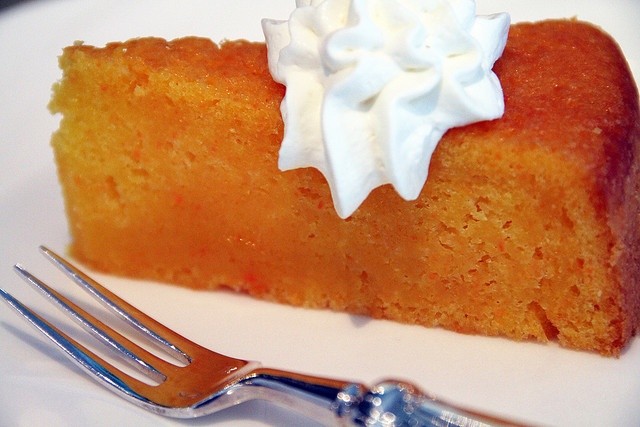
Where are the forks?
[0,244,533,427]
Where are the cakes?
[47,0,640,360]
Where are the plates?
[1,0,640,427]
